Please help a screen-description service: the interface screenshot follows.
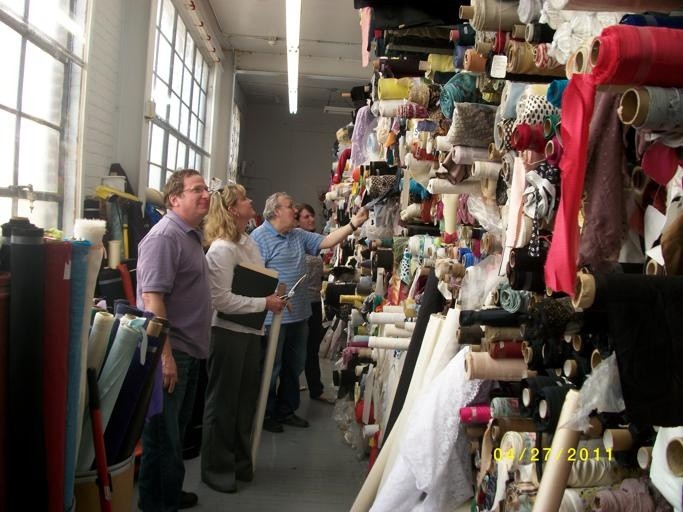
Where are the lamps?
[285,0,302,114]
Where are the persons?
[293,203,340,403]
[132,170,212,511]
[202,182,293,495]
[247,190,369,433]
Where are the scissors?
[280,274,307,305]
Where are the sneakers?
[264,411,310,432]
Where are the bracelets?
[347,217,357,231]
